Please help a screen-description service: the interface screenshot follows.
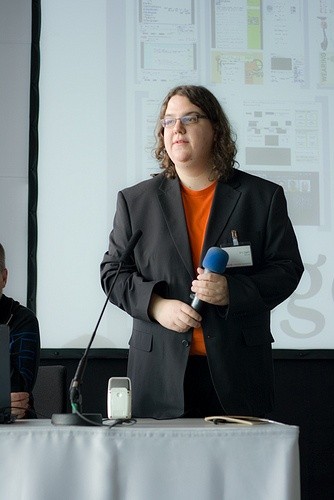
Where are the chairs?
[32,365,65,418]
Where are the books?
[204,416,269,426]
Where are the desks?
[0,417,300,500]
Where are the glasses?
[160,115,209,128]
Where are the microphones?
[51,230,143,426]
[192,248,230,312]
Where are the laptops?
[0,325,17,425]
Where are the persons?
[99,85,305,416]
[0,245,39,419]
